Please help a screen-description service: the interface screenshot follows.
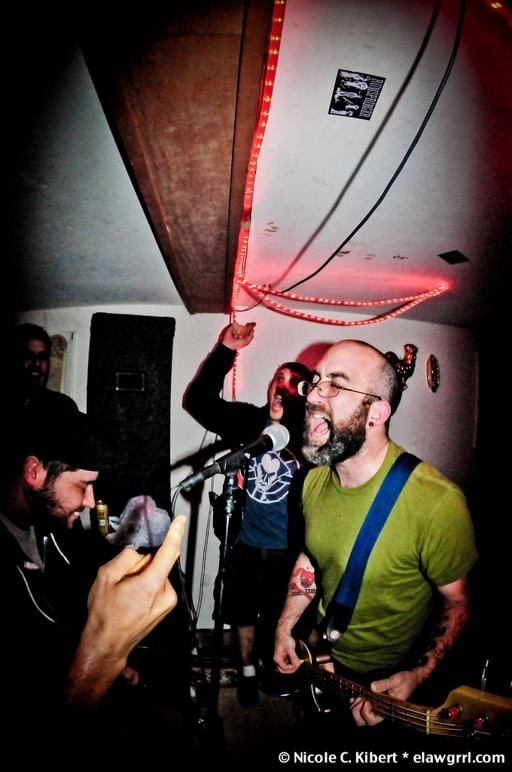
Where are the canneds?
[96,500,109,537]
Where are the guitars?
[254,627,511,741]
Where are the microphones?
[181,424,291,488]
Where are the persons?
[1,512,191,766]
[2,412,152,768]
[182,318,315,676]
[273,337,487,769]
[3,318,78,491]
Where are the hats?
[29,410,114,469]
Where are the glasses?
[296,380,382,401]
